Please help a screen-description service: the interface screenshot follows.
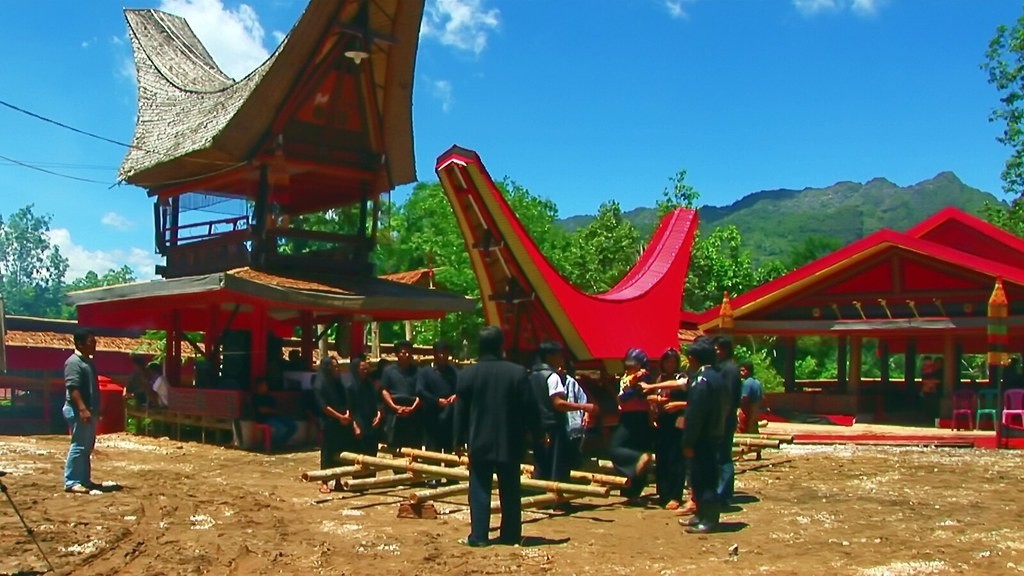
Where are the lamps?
[344,34,370,63]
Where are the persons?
[678,343,728,533]
[459,326,538,548]
[248,342,456,493]
[920,355,944,397]
[123,355,170,410]
[529,336,741,515]
[63,329,101,493]
[1004,356,1019,388]
[737,359,762,460]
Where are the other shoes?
[320,485,330,493]
[665,500,680,510]
[684,524,711,533]
[634,453,650,474]
[678,516,700,526]
[456,537,469,545]
[65,485,90,493]
[675,503,697,516]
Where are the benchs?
[128,385,241,446]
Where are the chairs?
[1002,389,1024,431]
[949,391,976,432]
[977,389,1000,433]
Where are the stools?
[251,423,272,453]
[306,417,322,447]
